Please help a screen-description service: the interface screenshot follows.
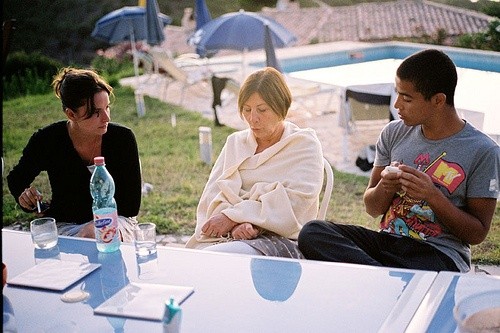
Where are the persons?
[7,65,143,245]
[183,65,324,260]
[296,48,500,272]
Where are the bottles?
[89,156,120,252]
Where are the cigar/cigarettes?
[35,196,41,214]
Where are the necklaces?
[66,121,99,166]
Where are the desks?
[405,271,500,333]
[2,229,439,333]
[341,82,398,162]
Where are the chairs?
[118,46,338,128]
[317,158,334,221]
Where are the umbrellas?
[262,22,281,75]
[189,8,298,84]
[194,0,220,62]
[90,6,173,117]
[145,0,166,103]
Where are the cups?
[135,222,156,256]
[452,288,500,333]
[30,217,58,250]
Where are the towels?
[185,121,324,250]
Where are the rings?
[212,233,217,237]
